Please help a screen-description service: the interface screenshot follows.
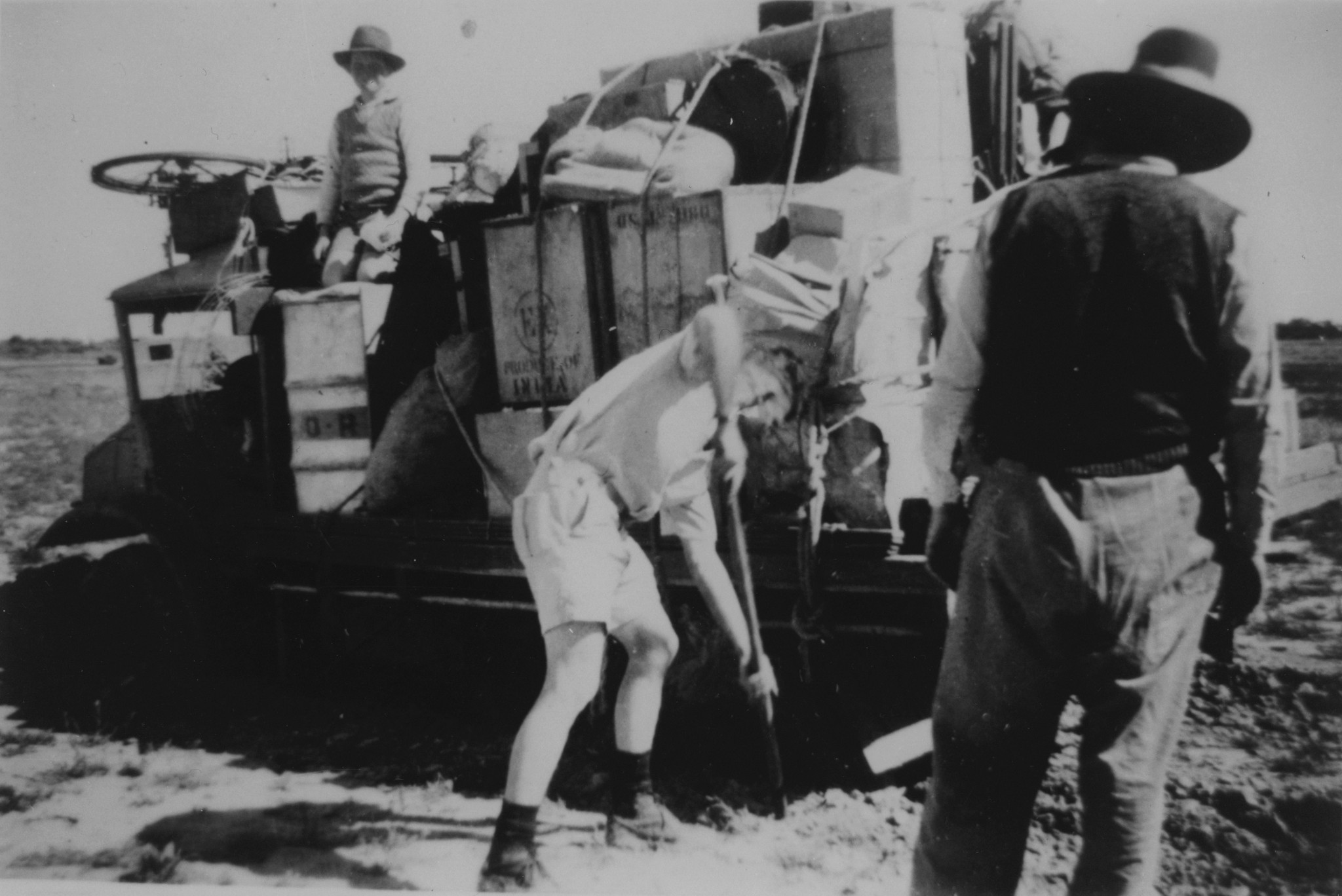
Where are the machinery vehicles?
[87,1,1342,652]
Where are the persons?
[312,25,431,285]
[475,299,803,893]
[908,27,1284,896]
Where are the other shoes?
[608,804,689,848]
[477,852,556,896]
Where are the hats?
[1066,26,1251,174]
[332,25,406,71]
